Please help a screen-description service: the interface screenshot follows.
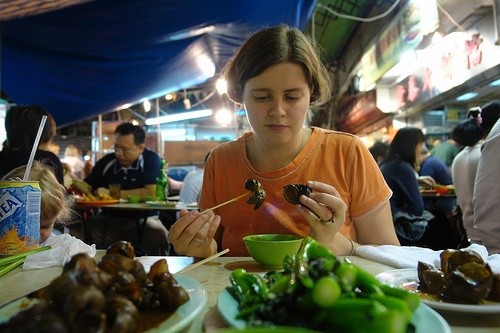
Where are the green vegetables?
[229,237,422,332]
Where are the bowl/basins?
[243,232,303,269]
[127,193,142,203]
[433,183,451,195]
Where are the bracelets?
[348,238,355,256]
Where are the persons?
[166,257,168,259]
[0,162,65,250]
[431,122,464,166]
[84,122,172,256]
[176,151,211,219]
[452,101,500,248]
[378,127,446,251]
[472,116,500,256]
[416,145,454,184]
[0,107,64,192]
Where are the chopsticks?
[0,244,51,266]
[197,189,252,216]
[0,258,25,276]
[170,248,231,278]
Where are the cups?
[109,183,122,202]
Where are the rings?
[320,216,336,224]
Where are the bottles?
[156,156,168,204]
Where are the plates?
[146,199,178,208]
[74,198,121,207]
[0,273,209,332]
[216,271,453,332]
[373,267,499,314]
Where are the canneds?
[0,180,41,256]
